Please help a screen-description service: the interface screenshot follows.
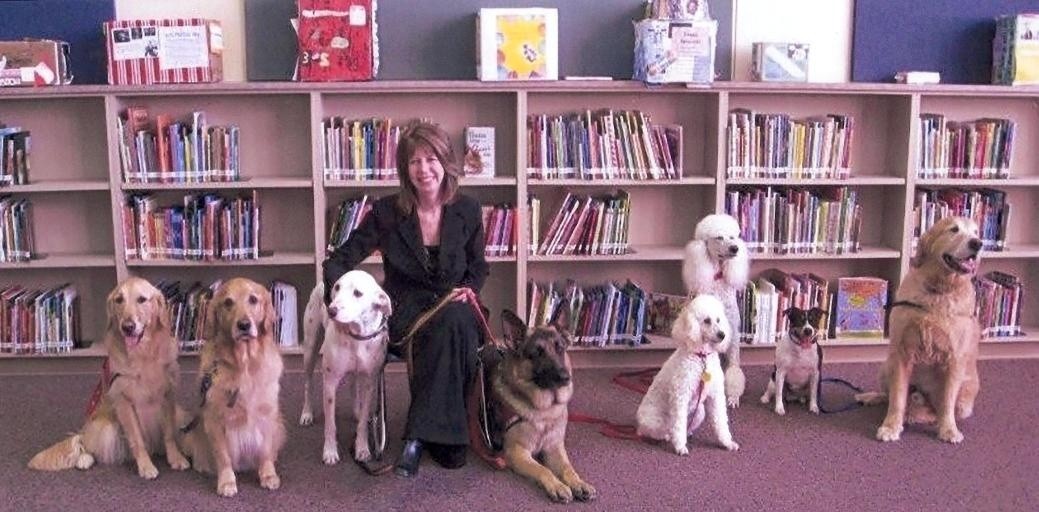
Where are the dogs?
[181,277,288,497]
[855,216,983,444]
[28,276,193,480]
[299,270,393,465]
[477,298,598,504]
[635,294,740,457]
[681,213,750,408]
[760,307,822,416]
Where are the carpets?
[1,358,1039,511]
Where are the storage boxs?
[105,20,222,85]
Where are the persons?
[321,124,490,477]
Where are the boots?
[394,438,423,477]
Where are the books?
[992,14,1039,85]
[0,123,32,186]
[1,282,79,355]
[122,190,262,259]
[527,108,689,347]
[321,114,517,258]
[912,113,1023,340]
[1,194,34,262]
[725,107,889,342]
[151,279,298,354]
[118,108,240,183]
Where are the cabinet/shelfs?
[0,79,1039,359]
